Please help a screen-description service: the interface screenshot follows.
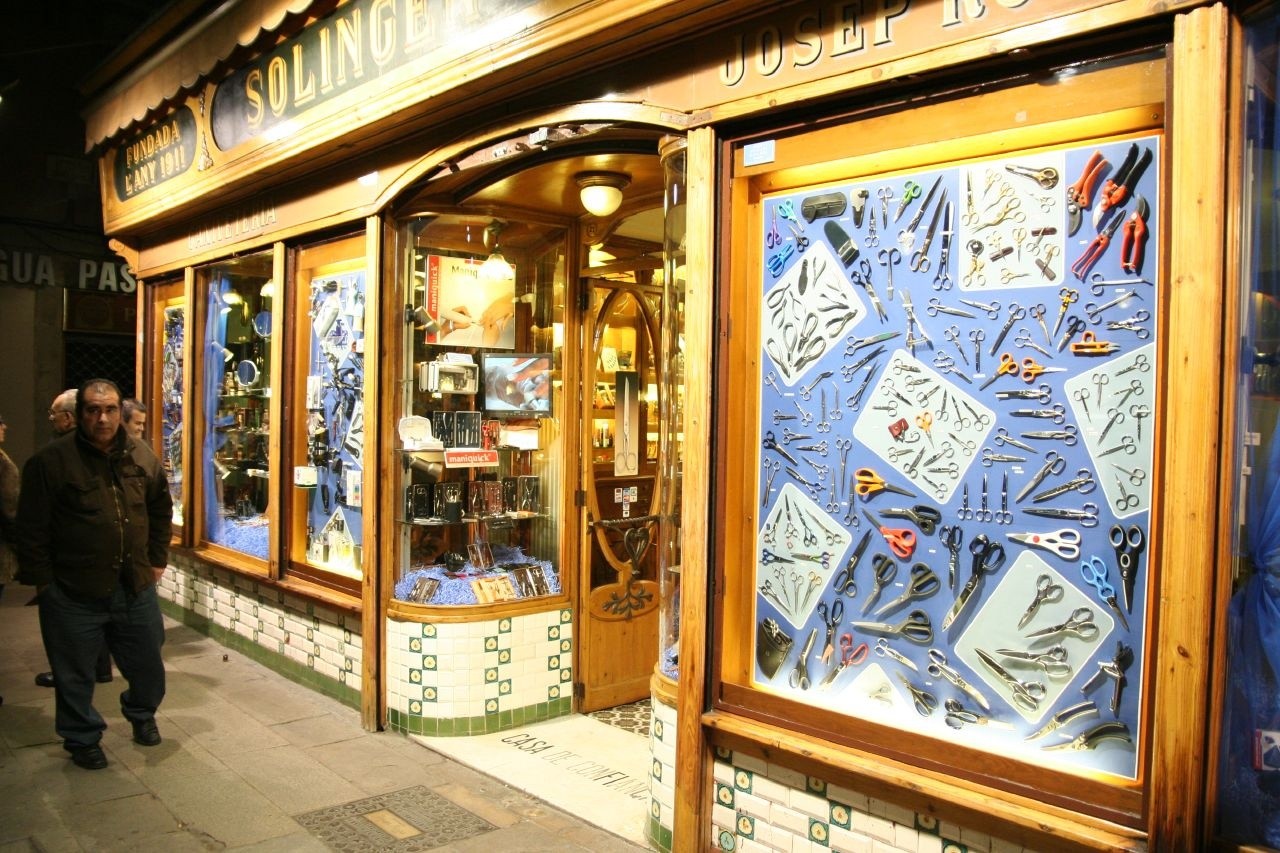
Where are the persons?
[13,380,174,769]
[432,293,515,346]
[0,388,149,685]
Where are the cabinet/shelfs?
[394,446,552,606]
[213,329,277,482]
[551,275,683,464]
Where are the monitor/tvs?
[480,351,557,420]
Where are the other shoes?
[121,708,162,745]
[35,672,56,688]
[25,597,39,606]
[95,663,114,683]
[63,739,107,769]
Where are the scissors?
[833,528,872,598]
[762,142,1154,752]
[939,524,963,589]
[849,608,934,643]
[615,377,637,473]
[1021,502,1100,528]
[861,509,916,558]
[957,482,974,520]
[995,470,1014,525]
[874,562,941,616]
[1032,468,1096,503]
[1005,528,1082,560]
[817,599,844,666]
[878,504,941,535]
[941,534,1005,632]
[974,648,1047,713]
[860,552,897,612]
[975,472,993,522]
[854,468,917,497]
[1015,450,1066,503]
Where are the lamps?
[212,271,233,315]
[221,287,244,306]
[574,172,633,219]
[209,340,235,363]
[259,278,273,298]
[477,221,515,280]
[404,304,444,338]
[209,457,232,481]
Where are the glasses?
[46,408,75,419]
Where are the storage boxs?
[295,466,319,490]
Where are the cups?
[618,350,632,369]
